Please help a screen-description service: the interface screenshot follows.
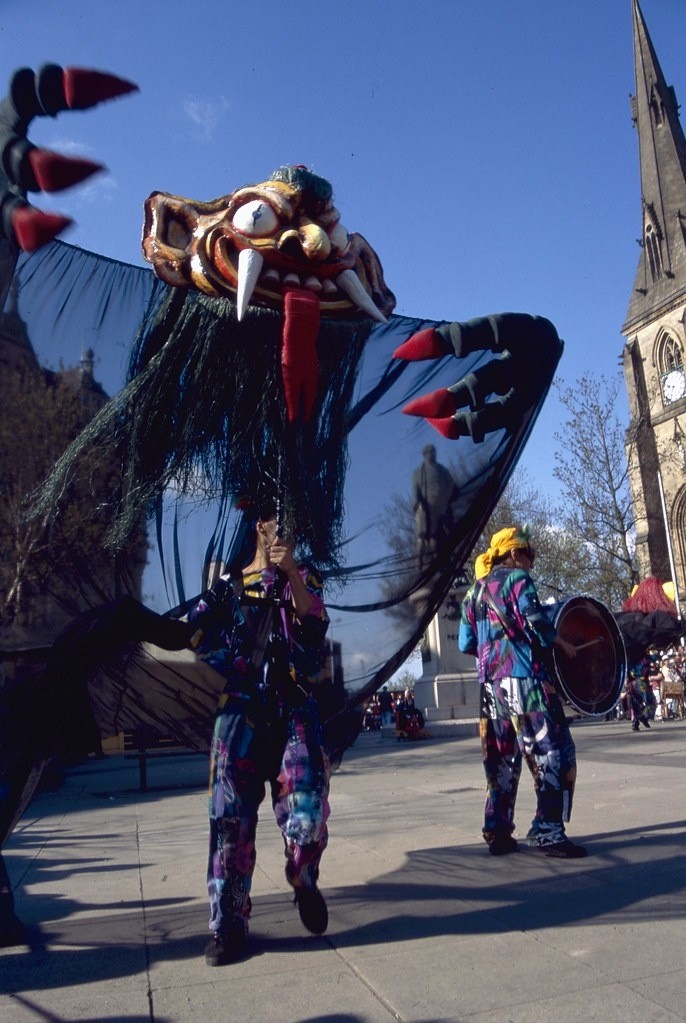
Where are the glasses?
[519,548,535,562]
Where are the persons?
[190,498,330,967]
[459,527,591,862]
[603,638,686,734]
[361,685,428,735]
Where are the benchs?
[124,716,214,792]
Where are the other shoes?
[632,726,640,731]
[639,716,650,727]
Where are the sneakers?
[204,913,256,966]
[292,884,329,933]
[489,837,522,853]
[544,840,588,858]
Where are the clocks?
[663,371,686,402]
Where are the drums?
[541,596,628,718]
